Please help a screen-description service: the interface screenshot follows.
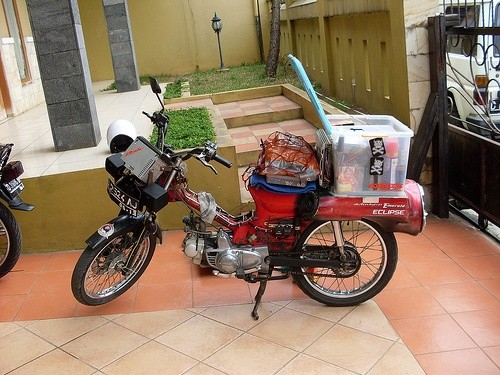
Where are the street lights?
[211,11,230,72]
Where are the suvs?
[438,0,500,141]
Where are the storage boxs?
[287,53,415,196]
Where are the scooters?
[0,143,35,281]
[71,76,427,321]
